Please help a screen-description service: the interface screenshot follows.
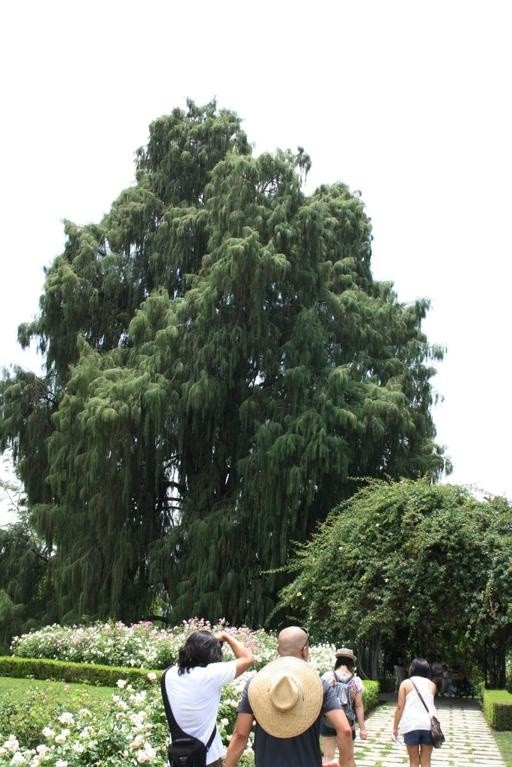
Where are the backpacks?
[323,672,356,729]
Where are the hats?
[336,648,357,661]
[248,655,323,738]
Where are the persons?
[160,630,257,766]
[390,657,440,766]
[393,654,476,700]
[223,625,356,766]
[321,647,369,766]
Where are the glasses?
[300,627,310,651]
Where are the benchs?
[453,672,470,697]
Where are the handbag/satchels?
[168,735,208,767]
[431,718,445,749]
